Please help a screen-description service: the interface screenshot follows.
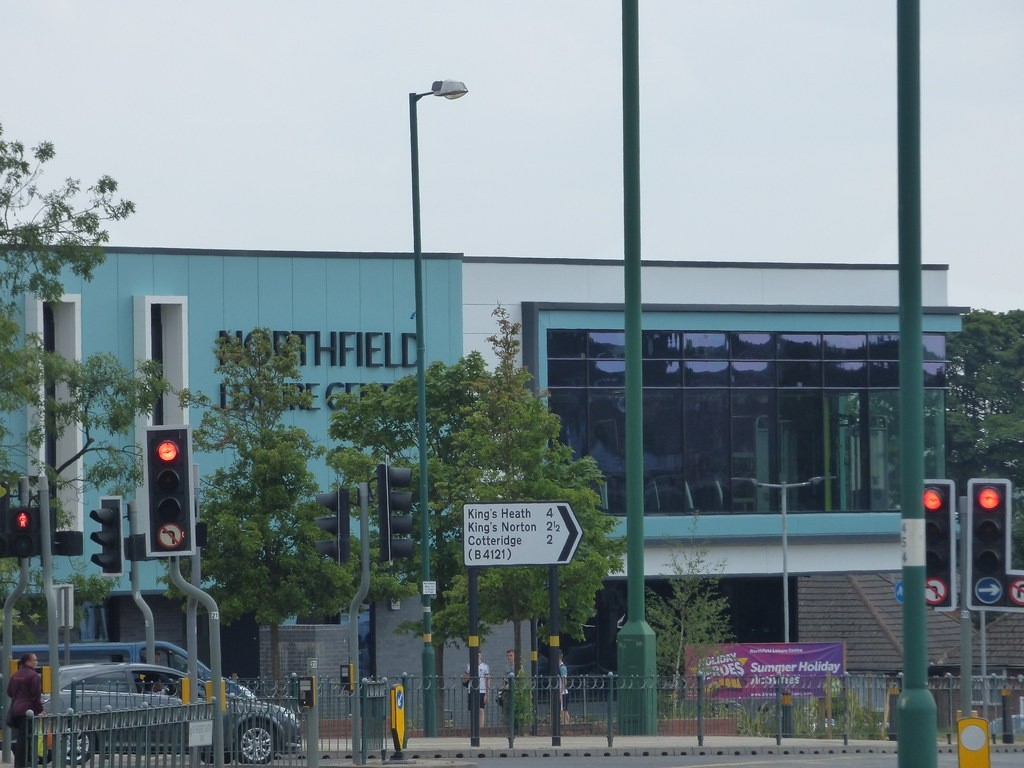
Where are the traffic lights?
[90,496,124,576]
[143,424,197,558]
[0,482,56,558]
[967,477,1010,613]
[378,456,415,563]
[922,479,956,614]
[315,485,351,567]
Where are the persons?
[7,653,43,768]
[463,651,491,727]
[143,675,162,694]
[499,650,524,708]
[560,650,570,724]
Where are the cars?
[36,662,301,765]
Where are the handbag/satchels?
[6,703,18,729]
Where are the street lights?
[408,80,470,743]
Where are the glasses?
[33,658,40,662]
[155,653,160,656]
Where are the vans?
[2,642,255,747]
